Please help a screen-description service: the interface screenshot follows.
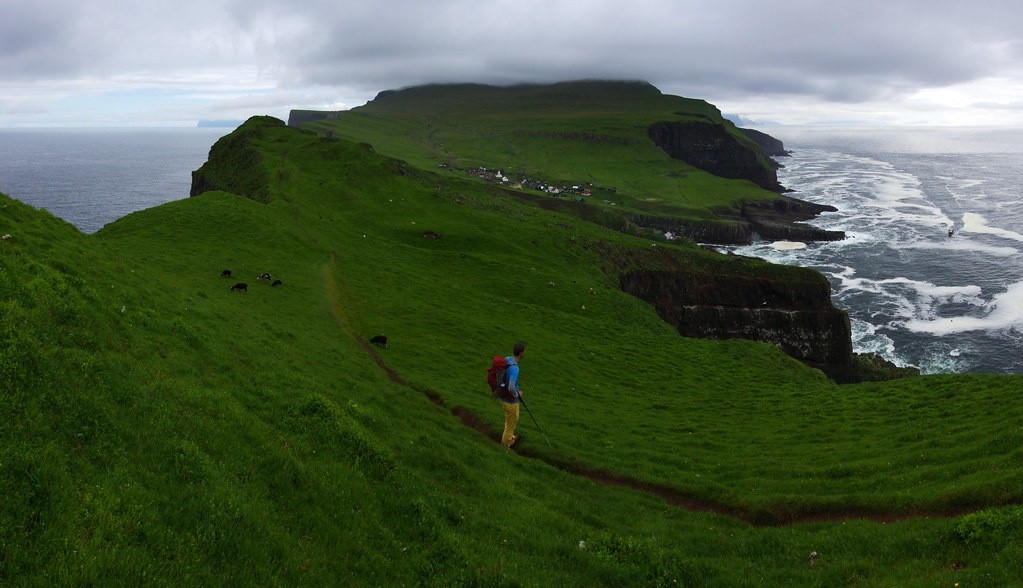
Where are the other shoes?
[510,433,520,447]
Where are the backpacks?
[485,355,515,401]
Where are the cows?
[369,336,388,349]
[220,269,231,279]
[270,280,282,287]
[256,273,272,280]
[230,282,248,292]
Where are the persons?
[485,343,525,448]
